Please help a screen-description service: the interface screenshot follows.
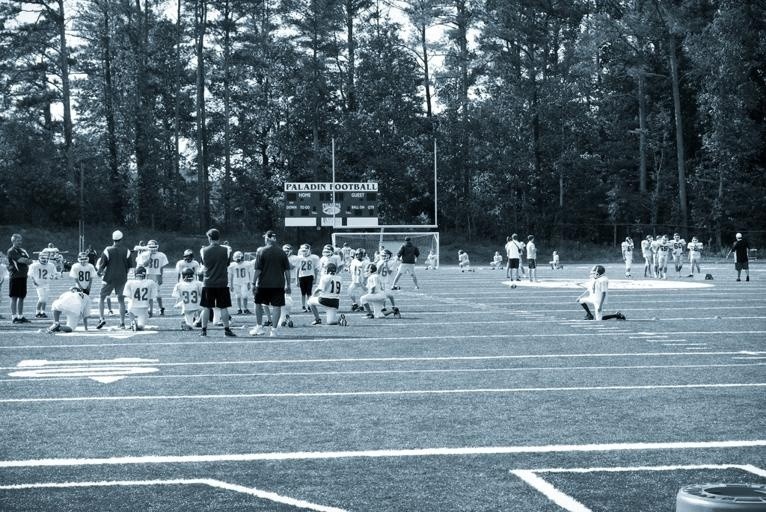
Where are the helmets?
[146,240,160,250]
[590,265,605,279]
[282,244,294,257]
[625,232,699,243]
[134,266,146,277]
[183,249,195,258]
[182,268,194,280]
[323,262,336,275]
[232,251,245,262]
[78,252,89,263]
[356,248,366,260]
[322,244,334,257]
[38,251,49,265]
[298,244,312,258]
[363,264,377,278]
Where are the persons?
[0,241,170,333]
[424,249,437,270]
[505,232,526,281]
[458,250,475,273]
[199,228,236,339]
[95,229,132,329]
[5,234,32,324]
[726,232,751,282]
[621,237,634,278]
[248,230,292,338]
[172,242,401,331]
[549,250,564,270]
[489,250,503,270]
[525,235,541,282]
[732,241,738,270]
[640,233,703,279]
[391,237,420,290]
[577,265,626,322]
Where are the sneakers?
[35,313,48,319]
[506,273,542,284]
[148,307,164,318]
[583,314,594,320]
[223,328,237,338]
[616,311,627,321]
[262,305,348,328]
[391,285,402,291]
[735,277,750,282]
[248,327,266,336]
[625,271,702,280]
[47,321,72,332]
[269,328,281,337]
[352,303,403,321]
[198,331,207,338]
[12,317,31,325]
[233,309,254,317]
[180,315,236,332]
[108,309,139,332]
[97,318,106,329]
